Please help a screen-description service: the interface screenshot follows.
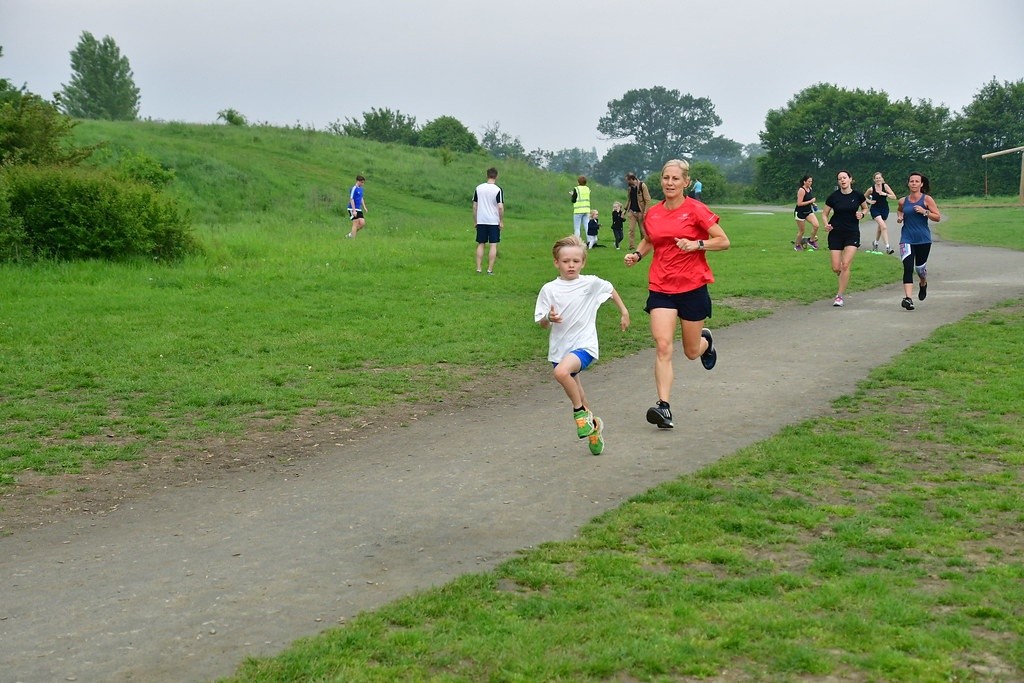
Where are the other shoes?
[486,271,493,275]
[345,233,351,239]
[476,270,482,273]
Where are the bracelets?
[925,210,929,216]
[633,252,642,262]
[545,312,552,322]
[624,208,628,212]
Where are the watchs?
[886,193,888,197]
[861,213,864,219]
[696,239,704,250]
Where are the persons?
[624,159,730,429]
[692,178,702,202]
[586,208,598,250]
[611,201,625,250]
[795,175,820,252]
[864,172,897,255]
[621,173,651,251]
[821,170,870,306]
[535,235,631,456]
[896,172,941,310]
[344,176,367,241]
[569,175,591,245]
[471,168,504,276]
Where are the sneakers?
[886,247,894,254]
[902,297,915,310]
[808,239,819,249]
[646,399,673,428]
[589,416,605,455]
[794,245,803,251]
[837,262,842,276]
[872,241,878,252]
[700,328,717,370]
[573,405,594,439]
[918,282,927,301]
[833,295,843,306]
